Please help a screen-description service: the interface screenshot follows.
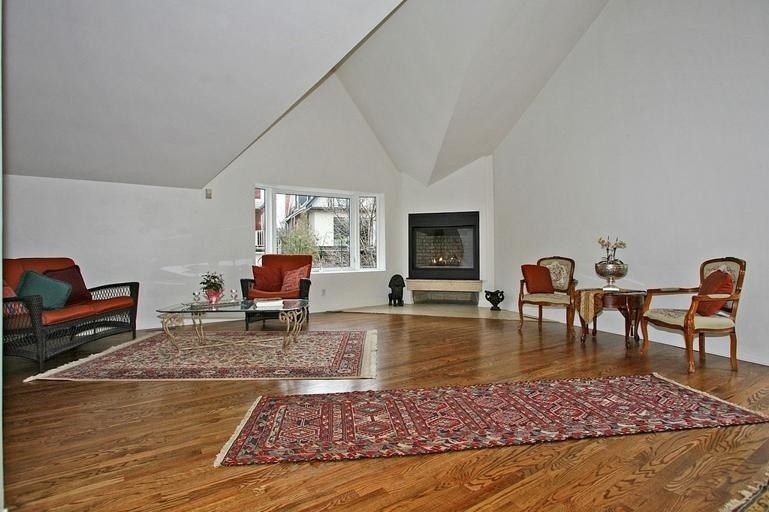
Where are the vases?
[595,262,627,291]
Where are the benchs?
[3,257,139,374]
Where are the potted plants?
[200,272,225,305]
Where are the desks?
[575,290,645,353]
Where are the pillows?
[15,270,73,310]
[3,280,29,316]
[695,270,734,317]
[281,264,311,291]
[44,264,92,306]
[521,264,554,293]
[251,265,282,291]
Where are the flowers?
[599,236,627,264]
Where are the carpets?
[214,373,769,467]
[21,328,378,383]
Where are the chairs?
[640,257,746,375]
[240,253,313,331]
[518,256,576,343]
